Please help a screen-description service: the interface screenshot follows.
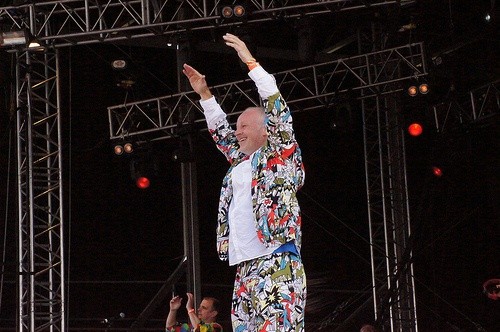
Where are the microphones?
[100,313,125,324]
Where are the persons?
[182,33,307,332]
[165,293,223,332]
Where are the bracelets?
[188,308,195,315]
[246,58,258,70]
